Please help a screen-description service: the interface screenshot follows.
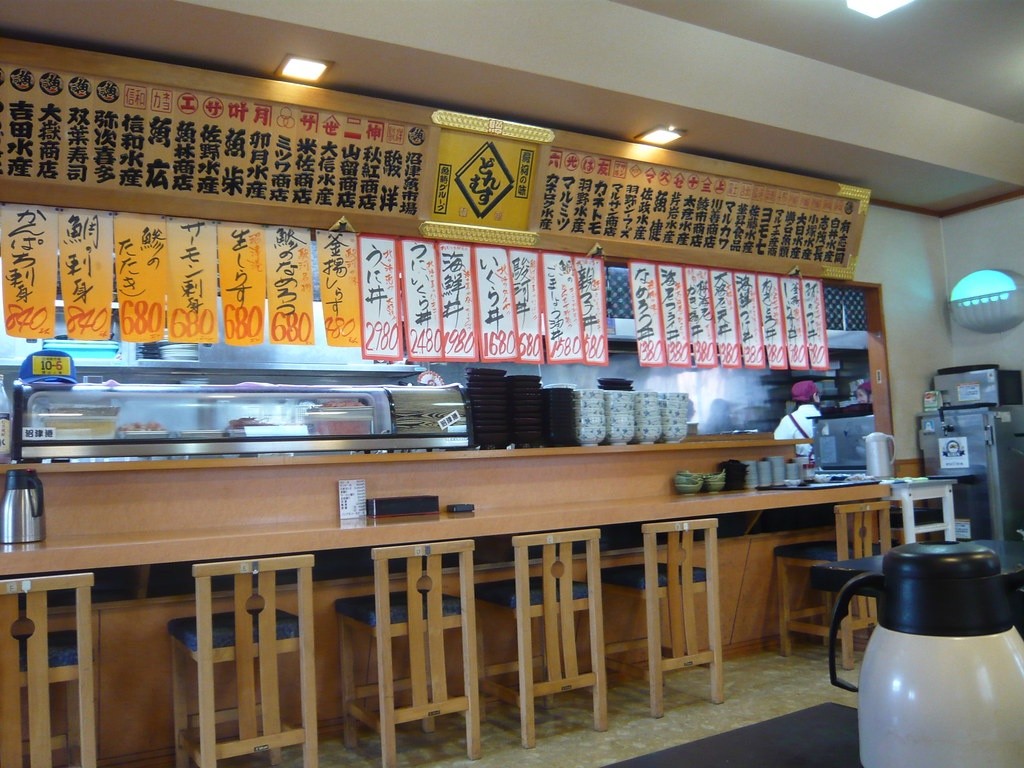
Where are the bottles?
[0,374,11,463]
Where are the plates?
[136,328,198,362]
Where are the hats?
[792,381,820,401]
[859,379,873,391]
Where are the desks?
[879,478,958,544]
[0,439,887,768]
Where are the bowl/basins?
[674,456,848,495]
[464,365,689,450]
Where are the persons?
[854,381,872,406]
[774,381,823,470]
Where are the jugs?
[863,432,896,477]
[0,468,47,544]
[829,542,1024,768]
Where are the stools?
[0,572,99,768]
[167,554,319,768]
[473,530,608,749]
[586,518,725,721]
[336,538,482,768]
[774,501,894,672]
